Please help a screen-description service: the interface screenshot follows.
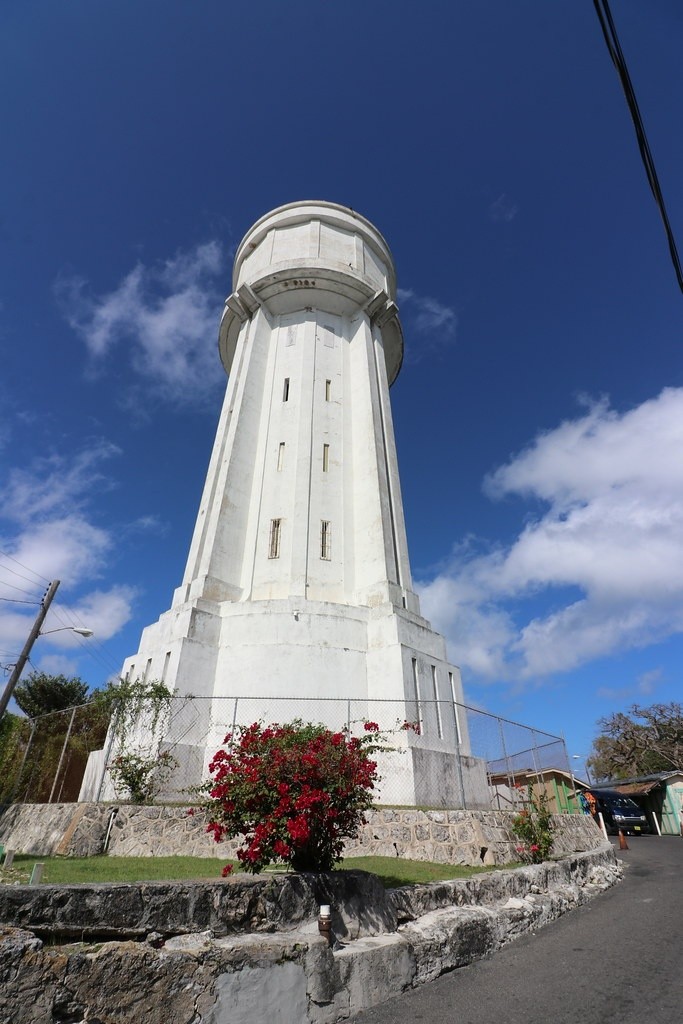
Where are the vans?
[584,790,649,835]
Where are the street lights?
[0,626,94,719]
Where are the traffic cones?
[617,831,631,851]
